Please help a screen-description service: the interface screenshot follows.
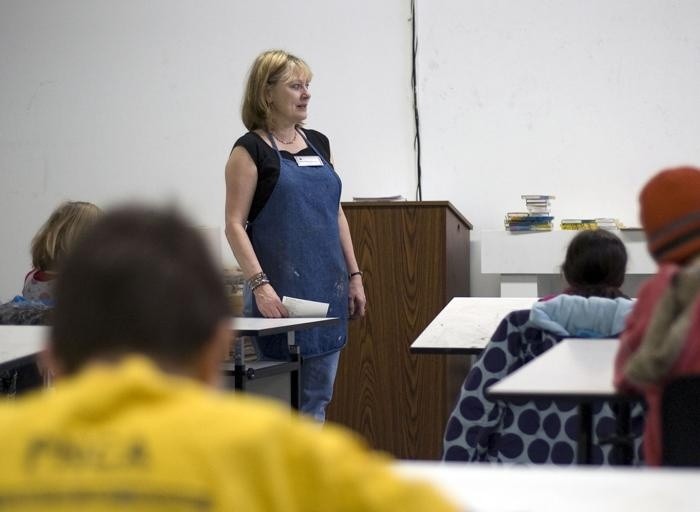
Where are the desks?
[406,296,544,354]
[0,325,57,390]
[485,338,632,464]
[480,230,657,298]
[405,458,699,511]
[219,317,340,414]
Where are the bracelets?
[349,270,363,280]
[247,271,271,291]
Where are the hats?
[640,167,700,266]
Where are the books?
[351,194,407,204]
[502,194,617,232]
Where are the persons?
[535,230,646,302]
[1,203,477,512]
[613,164,699,466]
[20,199,103,306]
[224,49,367,424]
